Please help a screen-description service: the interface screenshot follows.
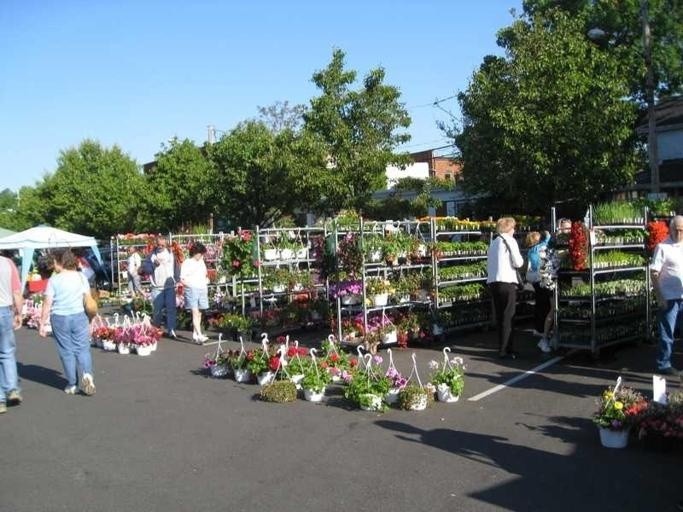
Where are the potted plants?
[433,199,678,358]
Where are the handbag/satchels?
[525,271,540,283]
[82,291,97,318]
[516,269,524,290]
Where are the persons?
[537,217,572,352]
[649,214,683,375]
[526,230,552,337]
[179,241,210,344]
[126,246,141,295]
[0,255,24,414]
[39,250,96,395]
[486,217,524,358]
[144,236,180,337]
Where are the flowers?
[22,297,48,332]
[205,347,463,412]
[88,311,159,359]
[644,393,682,442]
[114,218,432,346]
[591,380,652,426]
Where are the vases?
[598,426,632,450]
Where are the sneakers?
[64,385,76,394]
[536,338,550,353]
[80,372,96,396]
[656,366,679,375]
[192,333,209,345]
[0,400,6,414]
[7,388,22,402]
